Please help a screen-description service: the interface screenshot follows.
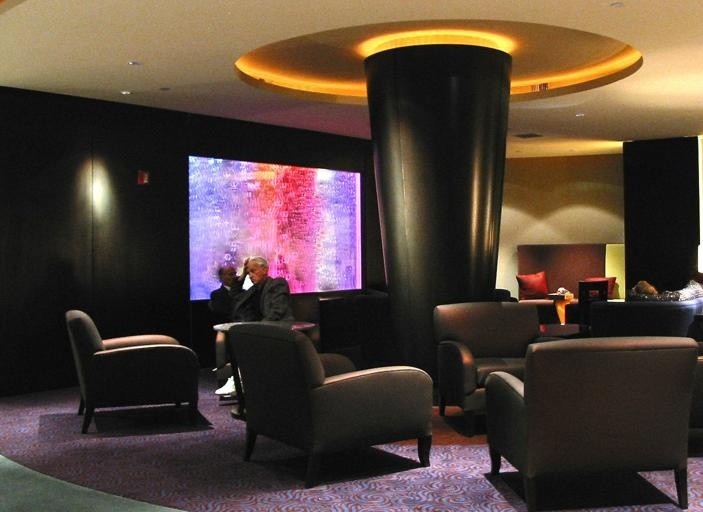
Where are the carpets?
[0,368,703,512]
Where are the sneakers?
[214,375,245,395]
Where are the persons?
[214,258,296,420]
[210,265,248,343]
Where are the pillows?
[582,276,617,299]
[516,270,549,299]
